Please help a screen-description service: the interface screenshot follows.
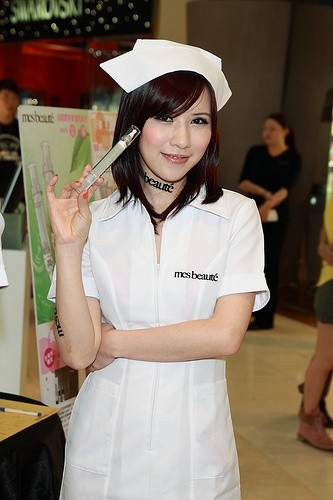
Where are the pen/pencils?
[0,407,42,418]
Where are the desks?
[0,391,66,500]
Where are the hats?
[99,39,232,112]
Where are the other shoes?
[297,404,333,451]
[247,320,275,330]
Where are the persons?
[47,38,271,500]
[0,79,28,243]
[297,185,333,452]
[239,113,303,330]
